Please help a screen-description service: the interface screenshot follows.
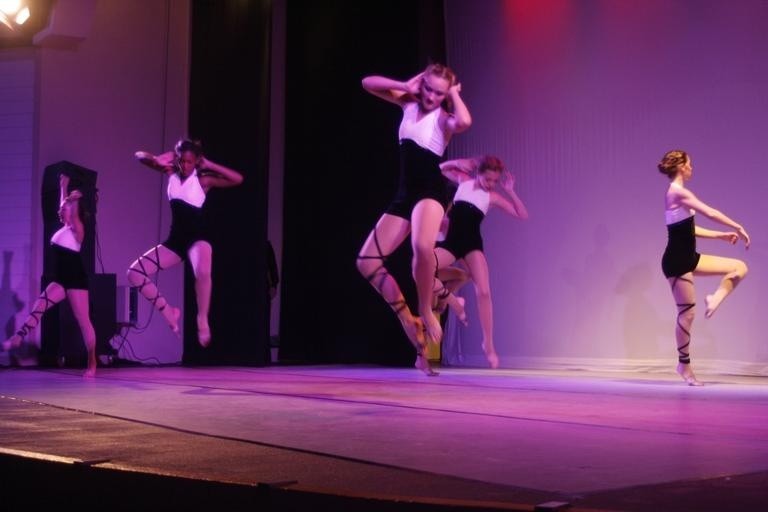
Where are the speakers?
[93,271,118,335]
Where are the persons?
[432,155,530,368]
[657,149,751,387]
[357,64,471,350]
[127,138,244,346]
[264,240,280,299]
[1,174,97,379]
[414,216,467,377]
